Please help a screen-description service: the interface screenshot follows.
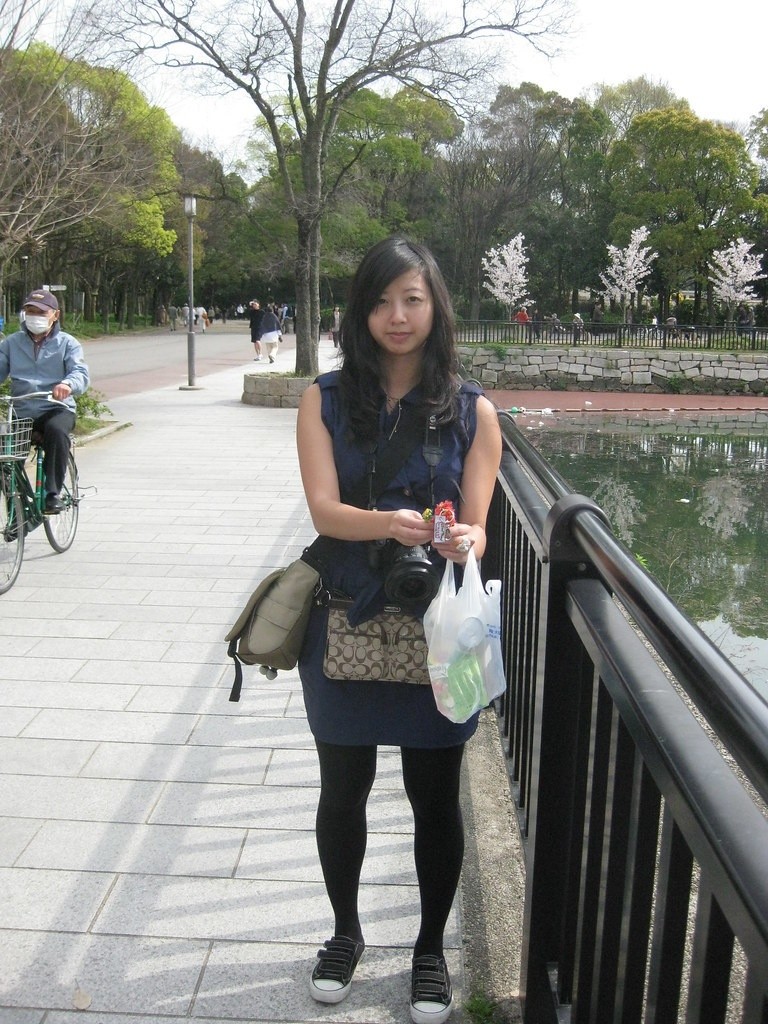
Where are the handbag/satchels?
[324,591,432,684]
[220,553,321,701]
[422,539,508,725]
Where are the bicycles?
[0,391,97,594]
[636,326,664,340]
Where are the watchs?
[60,380,71,388]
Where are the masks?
[24,312,57,335]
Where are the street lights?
[179,192,202,390]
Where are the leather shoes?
[4,516,19,542]
[45,495,66,512]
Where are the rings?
[454,538,471,552]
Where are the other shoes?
[254,358,261,361]
[268,355,274,364]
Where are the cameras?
[365,538,439,606]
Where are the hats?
[23,290,57,312]
[521,307,527,311]
[574,313,580,319]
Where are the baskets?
[0,418,33,462]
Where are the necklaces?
[378,376,410,437]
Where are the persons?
[159,299,344,364]
[296,237,503,1024]
[510,303,756,342]
[0,290,90,543]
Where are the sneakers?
[409,946,453,1024]
[309,931,365,1004]
